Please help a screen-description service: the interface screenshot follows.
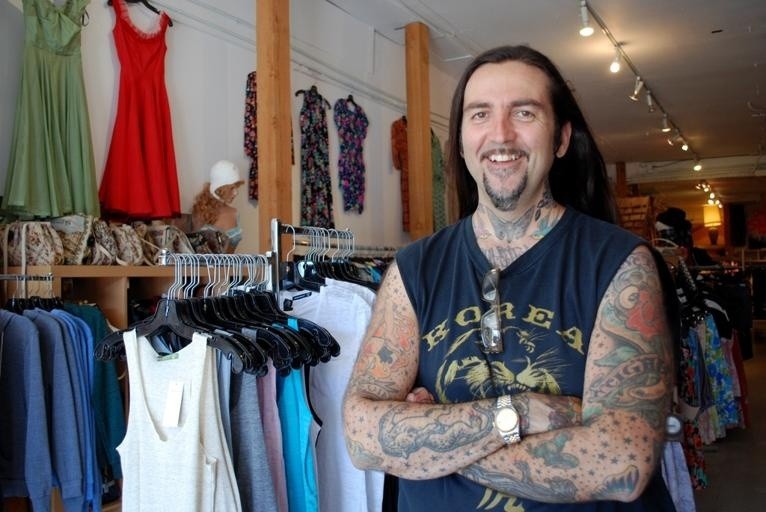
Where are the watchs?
[492,395,522,446]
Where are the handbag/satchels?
[3,213,227,267]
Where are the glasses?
[481,268,503,354]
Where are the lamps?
[578,1,701,171]
[703,204,723,246]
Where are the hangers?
[88,252,342,378]
[107,0,174,28]
[281,224,381,315]
[295,85,358,110]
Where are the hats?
[209,161,246,204]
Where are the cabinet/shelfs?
[3,260,262,512]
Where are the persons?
[187,160,246,254]
[339,41,682,512]
